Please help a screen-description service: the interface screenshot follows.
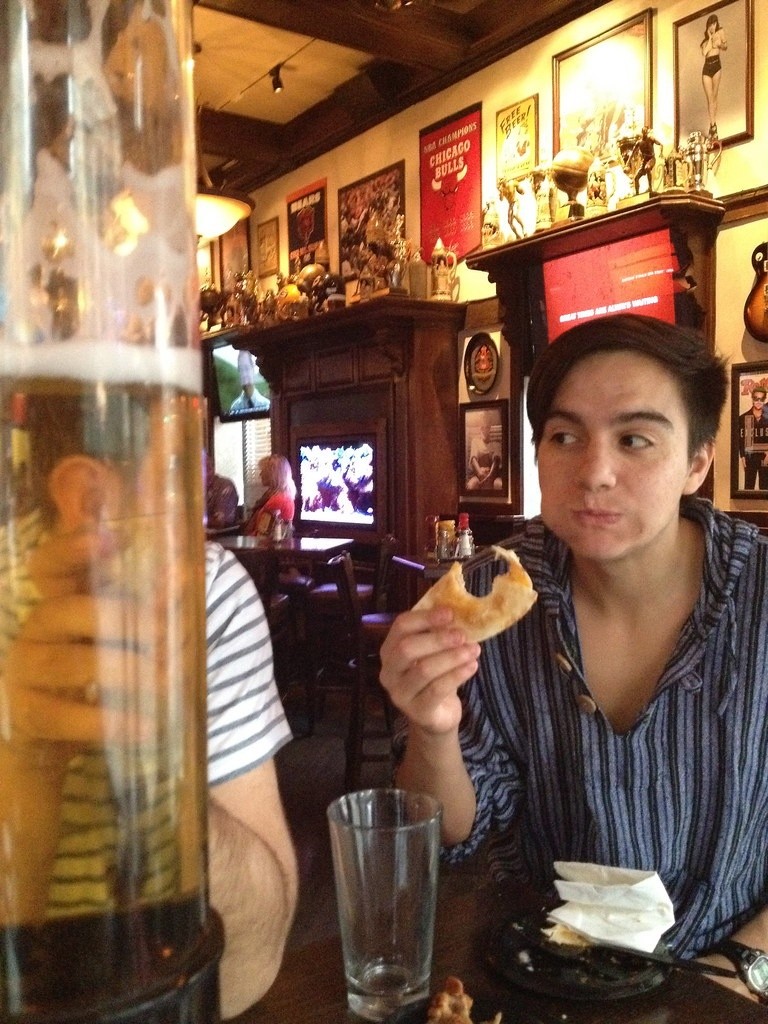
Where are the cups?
[327,786,442,1014]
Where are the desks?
[219,878,768,1024]
[391,552,476,578]
[213,534,357,561]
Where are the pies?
[411,544,538,644]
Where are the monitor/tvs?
[207,340,271,424]
[294,432,379,531]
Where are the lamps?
[197,187,256,245]
[270,64,283,94]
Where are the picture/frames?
[457,319,524,516]
[551,7,654,175]
[338,159,406,283]
[673,1,754,156]
[459,399,508,496]
[495,93,539,183]
[257,217,280,278]
[703,184,768,535]
[730,359,768,500]
[219,217,251,295]
[196,241,215,292]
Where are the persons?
[497,176,527,239]
[739,387,768,490]
[623,128,663,197]
[245,454,297,539]
[466,421,503,490]
[379,313,768,1005]
[205,454,238,524]
[0,390,293,1019]
[699,15,728,141]
[229,350,270,411]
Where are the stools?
[233,533,402,792]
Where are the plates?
[488,906,674,1002]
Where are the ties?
[248,400,254,408]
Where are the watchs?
[713,939,768,1000]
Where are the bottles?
[0,0,229,1024]
[456,513,474,558]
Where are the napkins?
[544,861,675,953]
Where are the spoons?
[512,917,738,980]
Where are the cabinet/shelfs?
[229,298,466,614]
